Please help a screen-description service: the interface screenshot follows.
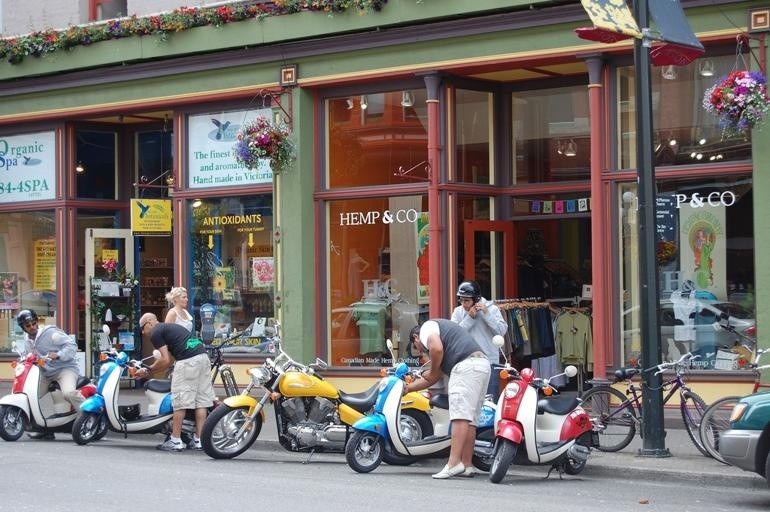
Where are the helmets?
[17,309,38,326]
[456,281,479,299]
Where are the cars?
[332,304,431,366]
[622,298,757,369]
[3,288,58,317]
[719,387,769,484]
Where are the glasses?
[139,322,147,331]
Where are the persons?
[136,313,213,450]
[164,287,193,332]
[451,281,507,401]
[16,309,87,440]
[404,318,493,479]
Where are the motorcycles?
[345,339,497,474]
[71,324,222,448]
[199,318,436,467]
[0,341,108,441]
[488,334,601,482]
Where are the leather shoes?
[432,463,464,480]
[456,466,475,478]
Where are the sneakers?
[156,436,184,453]
[187,433,203,451]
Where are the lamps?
[401,94,414,106]
[361,95,370,110]
[566,141,576,161]
[699,58,716,77]
[660,65,676,80]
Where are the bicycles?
[577,351,719,456]
[713,311,757,370]
[201,330,247,402]
[700,346,769,465]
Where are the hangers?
[488,294,597,315]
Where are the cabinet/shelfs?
[137,230,175,324]
